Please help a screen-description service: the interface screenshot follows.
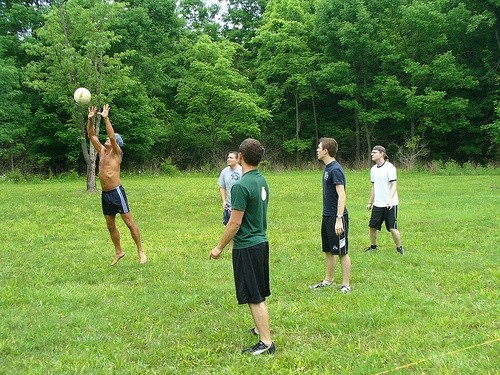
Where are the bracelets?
[367,203,372,204]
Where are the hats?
[115,133,123,146]
[373,145,389,160]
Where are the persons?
[87,104,147,266]
[364,146,404,255]
[313,138,351,294]
[209,139,276,353]
[218,151,242,253]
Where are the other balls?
[74,87,92,104]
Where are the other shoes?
[363,246,377,253]
[396,245,404,255]
[241,340,275,357]
[339,285,350,294]
[313,280,334,289]
[245,327,258,336]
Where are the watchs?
[336,215,343,219]
